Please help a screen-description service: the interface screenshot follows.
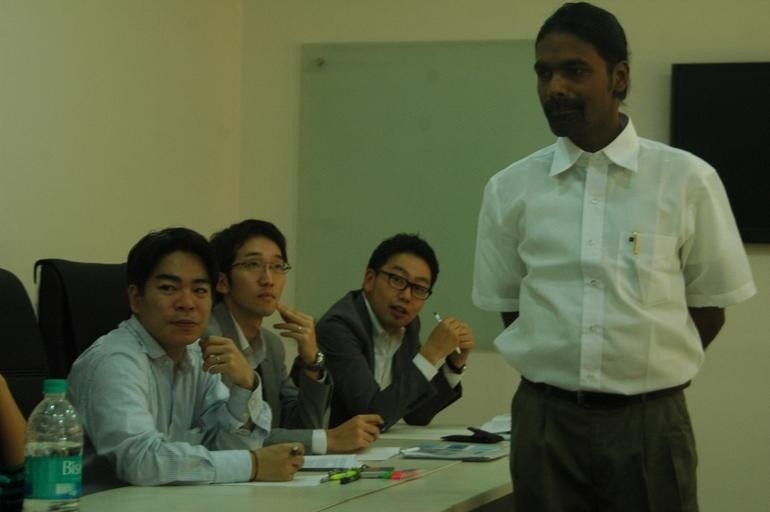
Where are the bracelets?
[250,449,260,481]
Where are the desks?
[22,426,512,512]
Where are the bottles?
[21,379,85,512]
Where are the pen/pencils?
[292,445,300,456]
[378,421,390,430]
[320,466,361,485]
[432,310,463,356]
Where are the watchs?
[445,354,467,374]
[306,351,327,372]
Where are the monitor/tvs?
[669,60,770,246]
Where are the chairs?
[0,269,52,423]
[33,259,144,384]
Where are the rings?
[292,444,300,455]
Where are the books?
[398,439,511,461]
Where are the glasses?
[230,257,292,273]
[379,264,431,302]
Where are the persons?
[472,3,756,509]
[199,218,385,455]
[0,370,35,507]
[66,228,305,494]
[289,233,474,432]
